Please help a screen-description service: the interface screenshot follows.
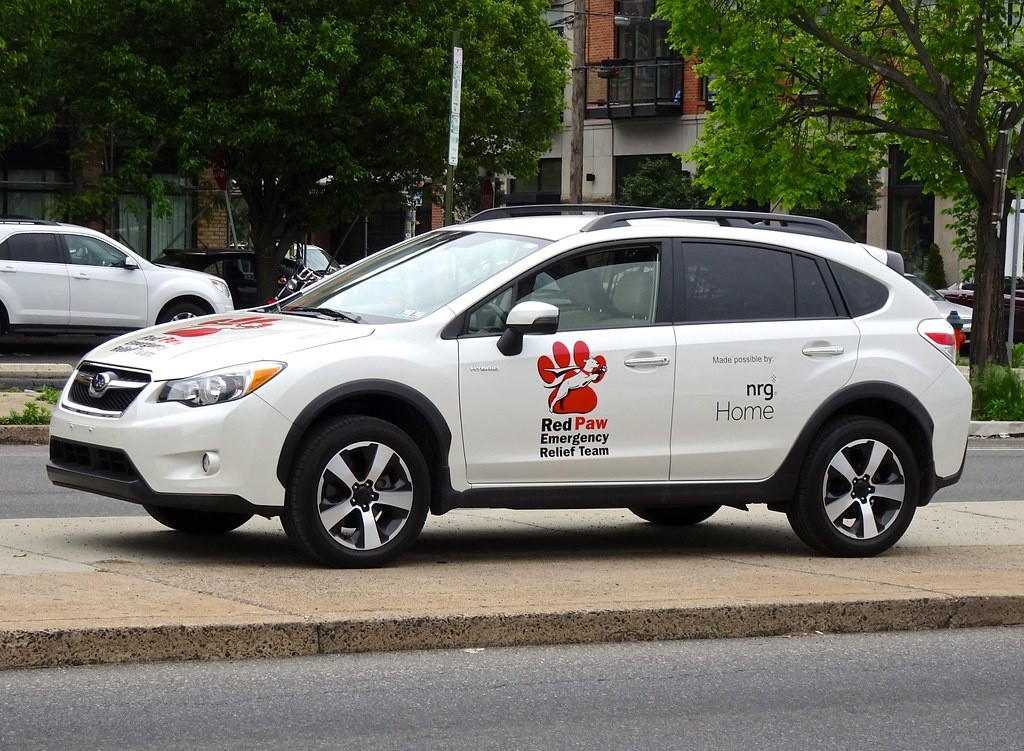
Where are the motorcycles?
[256,237,328,311]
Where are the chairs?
[585,269,653,329]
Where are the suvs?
[46,202,969,558]
[0,214,235,338]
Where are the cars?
[930,275,1024,331]
[230,241,348,281]
[150,236,327,312]
[903,273,973,347]
